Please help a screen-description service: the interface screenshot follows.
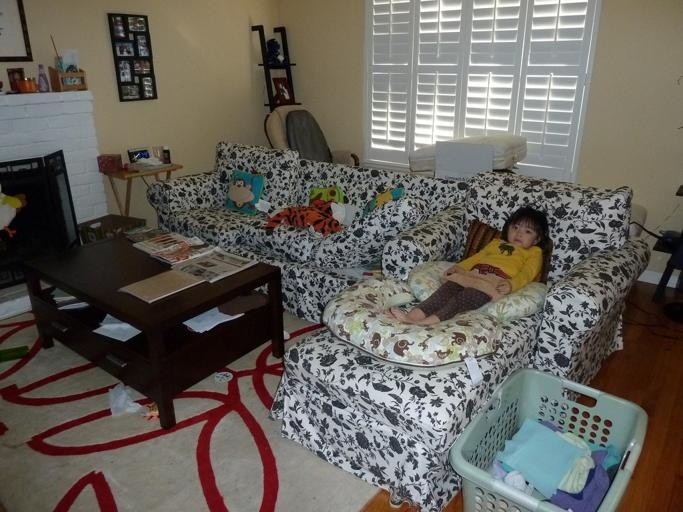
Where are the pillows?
[224,166,264,216]
[319,275,509,368]
[308,184,344,207]
[461,220,553,285]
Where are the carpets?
[0,309,382,512]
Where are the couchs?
[145,139,468,324]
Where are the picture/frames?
[106,12,158,102]
[0,0,33,63]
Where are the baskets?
[450,368,648,512]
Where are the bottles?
[162,146,169,164]
[19,77,36,93]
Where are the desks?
[102,160,184,218]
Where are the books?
[122,225,257,284]
[117,270,206,303]
[51,287,89,311]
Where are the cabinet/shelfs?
[250,25,301,113]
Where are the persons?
[381,206,549,328]
[123,63,129,84]
[121,46,130,54]
[282,86,290,101]
[277,82,287,103]
[139,38,148,55]
[65,65,79,87]
[265,38,283,68]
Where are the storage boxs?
[74,214,146,246]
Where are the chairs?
[378,170,650,428]
[262,101,359,167]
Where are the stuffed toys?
[265,196,343,237]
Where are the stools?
[268,313,533,511]
